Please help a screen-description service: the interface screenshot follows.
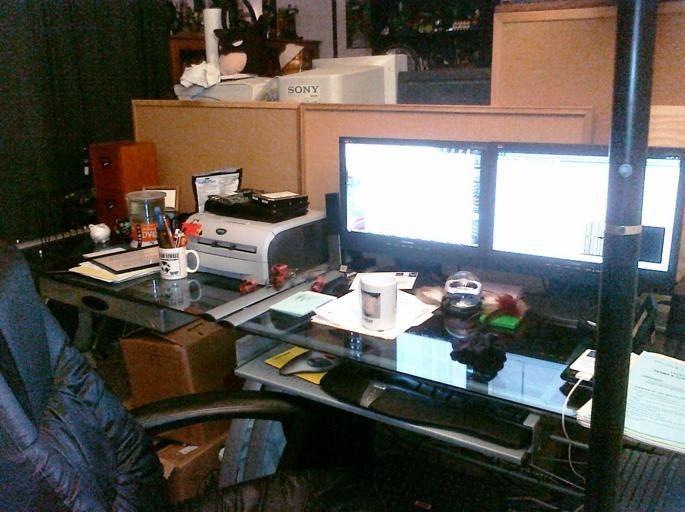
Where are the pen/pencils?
[154,206,190,248]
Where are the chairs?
[0,240,405,512]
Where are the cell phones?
[563,350,596,391]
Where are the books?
[68,244,160,284]
[270,290,338,318]
[174,78,276,104]
[575,348,685,453]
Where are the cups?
[125,191,167,249]
[157,247,200,281]
[361,271,398,331]
[358,334,398,379]
[158,277,203,311]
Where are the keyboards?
[319,359,538,448]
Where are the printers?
[182,209,328,286]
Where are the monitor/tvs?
[350,317,596,432]
[489,140,685,324]
[338,135,487,277]
